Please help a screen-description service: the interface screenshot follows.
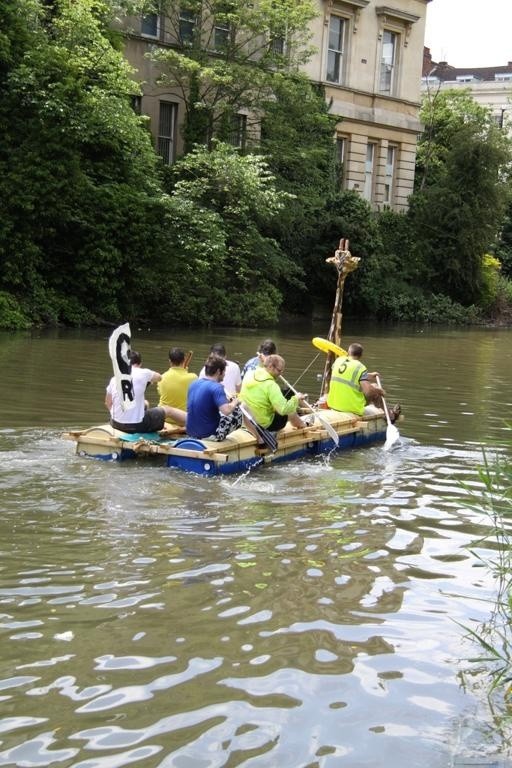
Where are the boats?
[59,230,389,483]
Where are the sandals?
[384,404,402,424]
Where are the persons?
[104,350,187,434]
[240,354,316,432]
[157,347,199,425]
[199,343,242,397]
[327,343,401,425]
[185,356,277,449]
[240,340,309,408]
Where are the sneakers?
[304,414,315,427]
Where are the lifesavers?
[313,336,347,357]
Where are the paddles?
[375,376,400,440]
[224,388,278,454]
[279,376,339,445]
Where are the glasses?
[272,364,284,374]
